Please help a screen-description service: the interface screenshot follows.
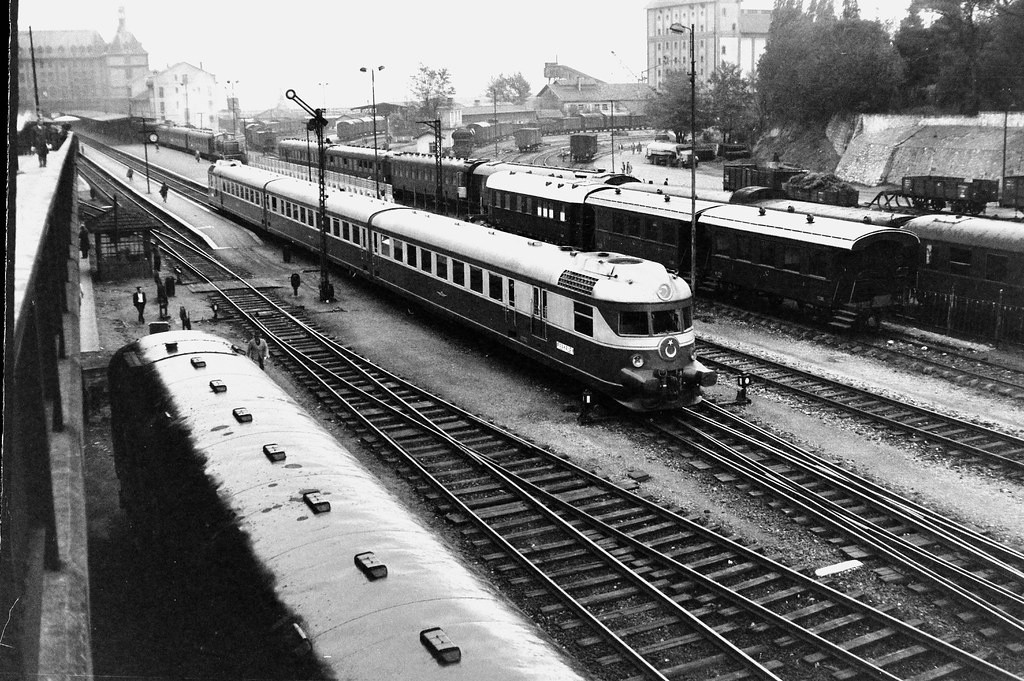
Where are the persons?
[126,166,133,181]
[195,148,200,163]
[79,226,192,330]
[159,182,168,202]
[291,272,301,296]
[36,141,49,167]
[212,302,270,370]
[619,142,642,174]
[664,178,669,185]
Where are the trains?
[207,159,719,415]
[512,128,543,153]
[278,138,1024,339]
[238,113,389,153]
[450,112,653,161]
[131,120,247,164]
[569,133,597,164]
[108,318,598,681]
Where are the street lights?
[360,65,386,200]
[669,23,695,317]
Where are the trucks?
[646,140,699,168]
